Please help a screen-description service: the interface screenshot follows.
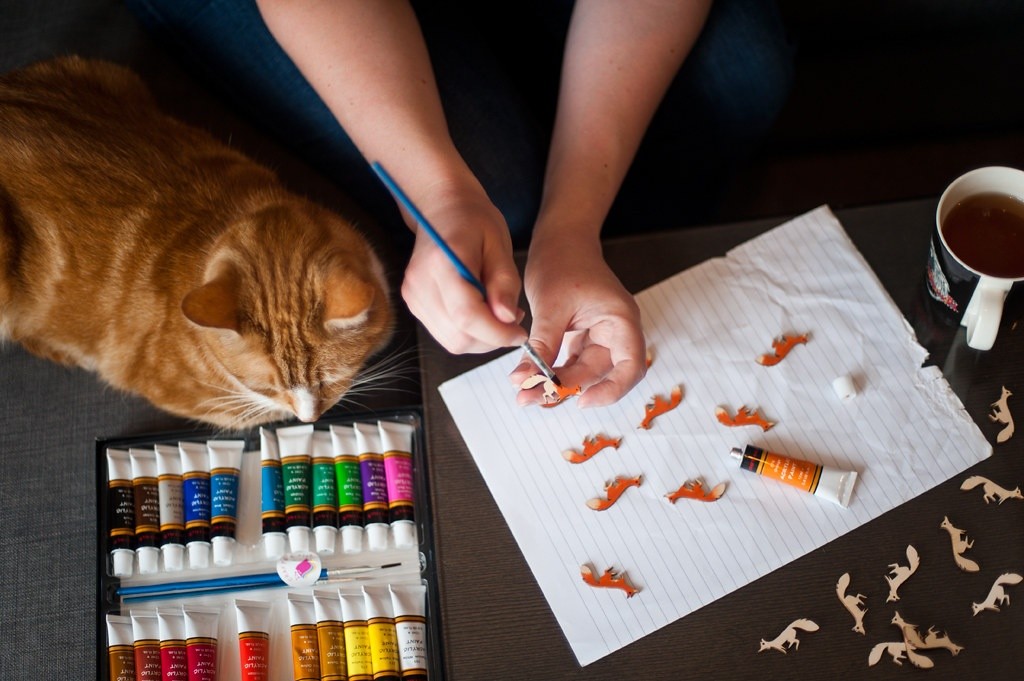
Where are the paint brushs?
[117,562,403,604]
[373,162,562,388]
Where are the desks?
[407,203,1024,681]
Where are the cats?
[0,52,398,437]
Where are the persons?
[127,0,794,406]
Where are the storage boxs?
[93,407,446,681]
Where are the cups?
[920,164,1024,350]
